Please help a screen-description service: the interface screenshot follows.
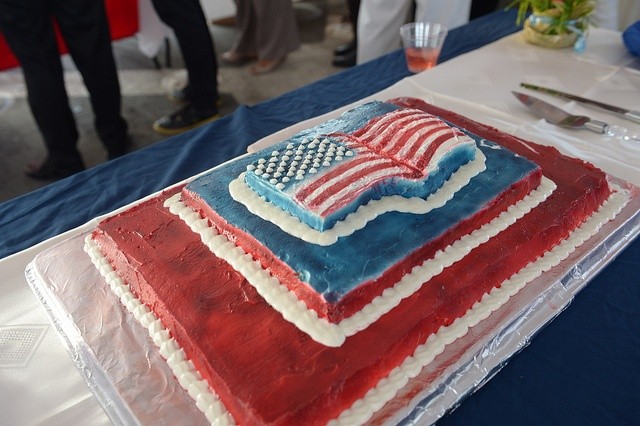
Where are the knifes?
[520,80,640,123]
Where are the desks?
[0,0,640,426]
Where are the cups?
[400,22,448,73]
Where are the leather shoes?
[331,50,356,67]
[21,160,45,180]
[251,56,286,75]
[166,88,223,107]
[152,103,220,135]
[333,42,356,56]
[219,47,253,68]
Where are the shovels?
[511,91,640,150]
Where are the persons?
[220,1,300,76]
[3,2,138,179]
[332,1,357,67]
[357,0,473,65]
[151,0,222,134]
